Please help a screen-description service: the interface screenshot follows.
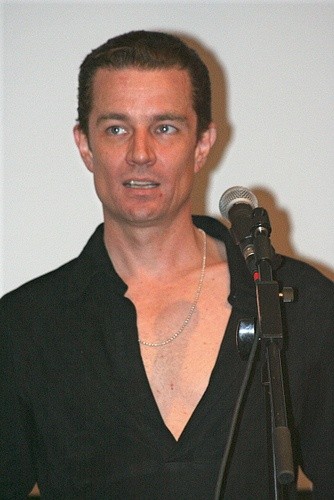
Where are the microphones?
[218,186,262,281]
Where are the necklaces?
[138,229,207,347]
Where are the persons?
[1,31,334,500]
